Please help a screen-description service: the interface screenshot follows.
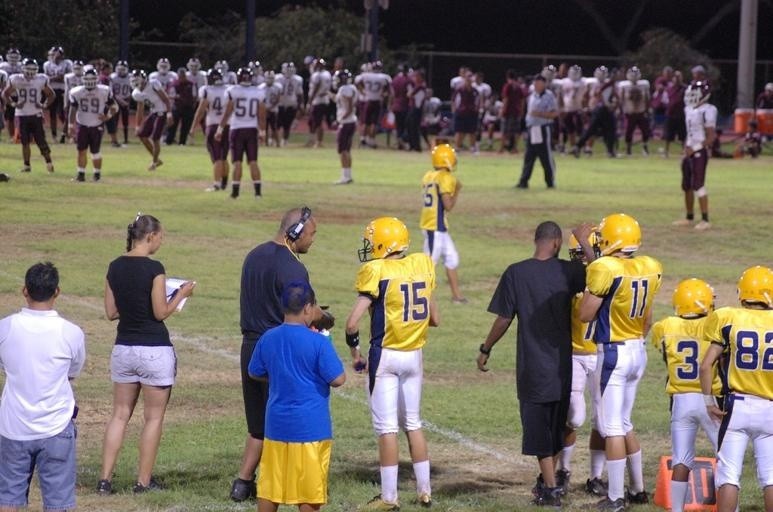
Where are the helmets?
[592,212,643,257]
[48,47,64,60]
[566,65,583,82]
[540,64,559,82]
[592,65,610,82]
[567,226,600,263]
[357,216,411,263]
[21,57,39,70]
[431,143,460,171]
[671,276,718,318]
[624,65,643,81]
[71,57,413,86]
[735,265,773,310]
[5,49,21,61]
[682,78,712,109]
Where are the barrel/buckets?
[734,108,754,134]
[755,109,773,135]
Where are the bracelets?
[346,328,360,347]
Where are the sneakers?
[229,476,258,503]
[360,493,402,512]
[449,294,473,305]
[624,488,650,507]
[411,491,433,509]
[584,476,608,498]
[204,184,220,193]
[333,178,353,186]
[671,218,712,232]
[527,469,572,511]
[95,478,114,497]
[145,157,164,173]
[578,496,627,512]
[131,479,168,497]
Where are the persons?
[346,217,441,511]
[248,282,345,511]
[95,211,197,497]
[230,203,336,504]
[419,144,469,304]
[1,45,773,231]
[476,213,772,511]
[1,260,86,510]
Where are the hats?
[464,71,476,83]
[526,74,548,83]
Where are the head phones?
[286,207,311,242]
